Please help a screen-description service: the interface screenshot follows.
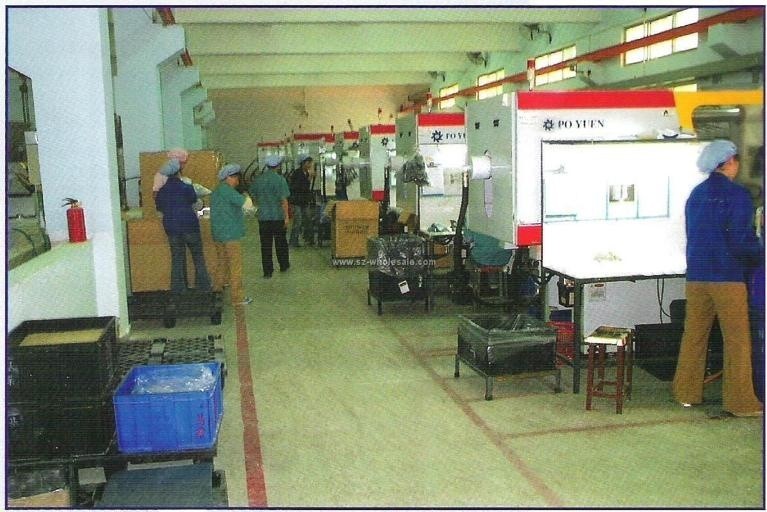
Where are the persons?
[248,151,292,278]
[209,161,253,306]
[150,146,190,200]
[152,158,210,291]
[287,152,319,249]
[668,136,763,417]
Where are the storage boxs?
[323,201,379,258]
[9,316,224,460]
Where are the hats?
[265,154,283,167]
[295,153,310,165]
[159,157,182,176]
[168,151,187,162]
[696,139,738,175]
[217,162,241,181]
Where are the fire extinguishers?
[60,198,86,242]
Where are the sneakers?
[239,296,253,305]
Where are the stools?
[584,327,634,413]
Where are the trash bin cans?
[545,321,576,369]
